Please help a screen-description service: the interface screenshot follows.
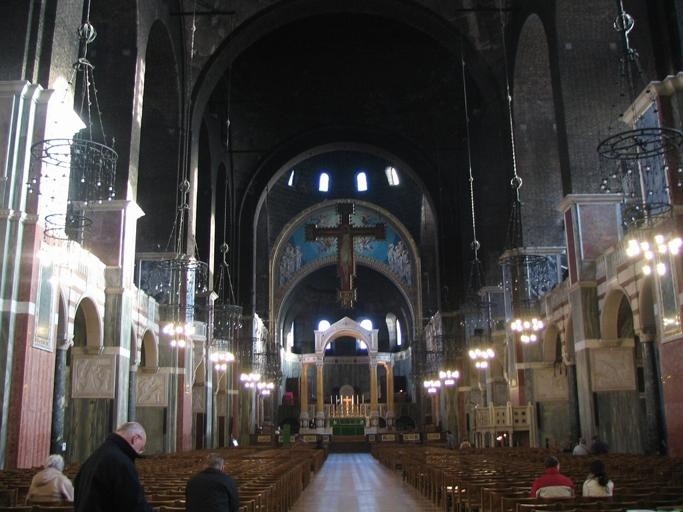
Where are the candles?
[330,393,364,405]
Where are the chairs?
[0,447,325,512]
[371,445,683,512]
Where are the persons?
[528,455,575,499]
[571,437,590,457]
[459,436,470,449]
[137,376,155,404]
[588,432,609,456]
[445,431,458,450]
[183,450,240,511]
[68,421,155,512]
[25,453,74,507]
[76,358,111,394]
[228,432,236,448]
[581,459,614,497]
